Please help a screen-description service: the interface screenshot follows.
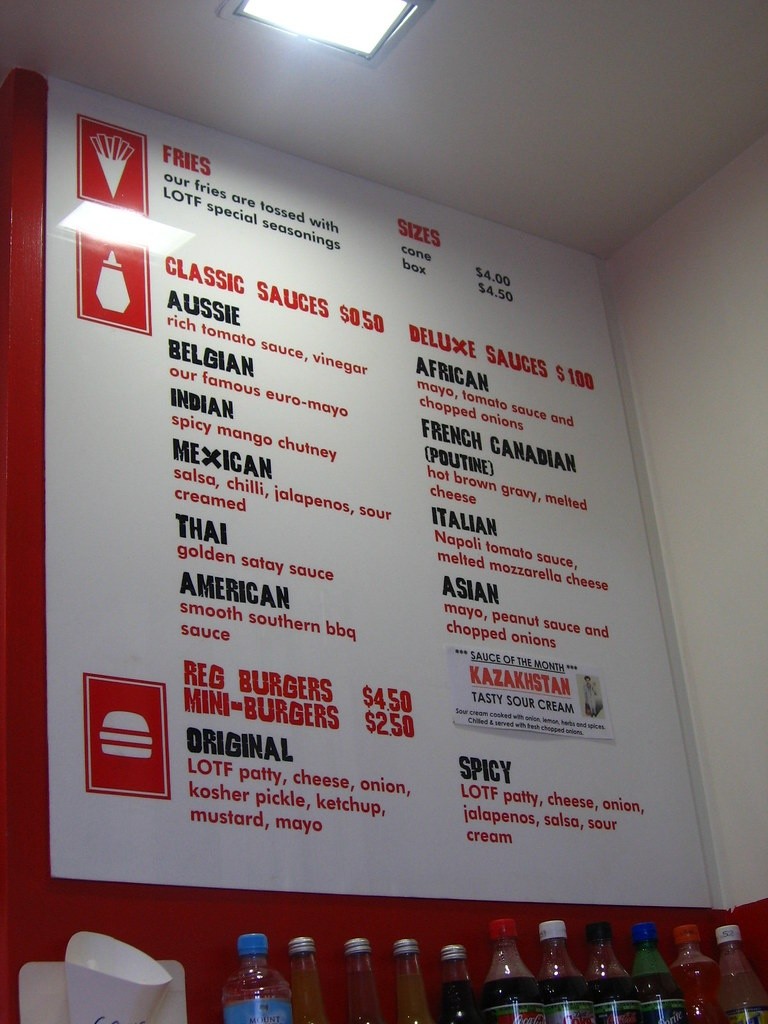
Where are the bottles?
[535,920,597,1024]
[392,938,436,1024]
[630,922,688,1024]
[583,921,641,1024]
[438,941,479,1024]
[670,923,732,1024]
[713,924,768,1024]
[287,935,332,1024]
[221,931,295,1024]
[343,936,386,1024]
[479,919,548,1024]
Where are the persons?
[584,676,598,717]
